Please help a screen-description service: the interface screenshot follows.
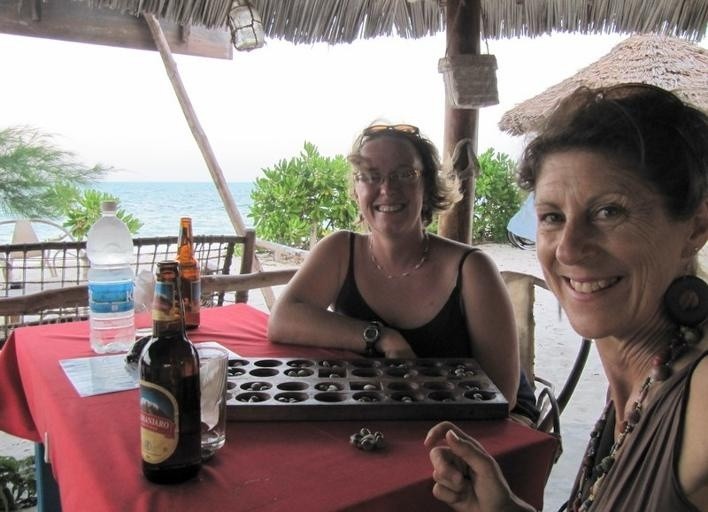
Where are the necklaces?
[562,326,702,511]
[367,228,430,280]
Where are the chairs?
[501,269,593,465]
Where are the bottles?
[176,217,199,330]
[137,259,200,486]
[85,199,136,354]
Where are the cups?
[193,346,228,456]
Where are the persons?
[265,120,540,434]
[420,81,706,512]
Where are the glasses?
[559,82,705,176]
[354,168,421,185]
[358,125,425,148]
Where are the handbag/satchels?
[437,54,498,106]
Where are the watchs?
[360,321,383,351]
